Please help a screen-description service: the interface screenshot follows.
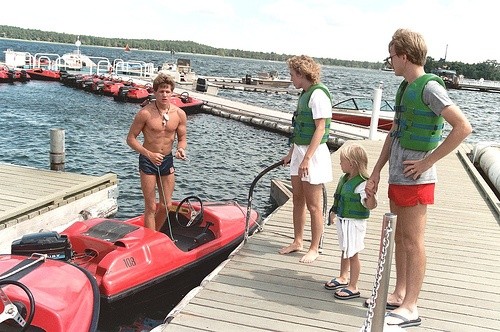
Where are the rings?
[410,168,414,174]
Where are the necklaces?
[154,100,171,127]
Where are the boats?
[251,73,292,88]
[0,254,100,332]
[332,98,396,131]
[46,196,261,304]
[0,65,205,110]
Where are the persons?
[366,28,473,328]
[278,55,334,264]
[325,143,377,300]
[126,72,188,231]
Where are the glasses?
[384,52,397,66]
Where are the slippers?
[325,278,349,290]
[385,311,422,328]
[365,293,399,310]
[334,288,360,299]
